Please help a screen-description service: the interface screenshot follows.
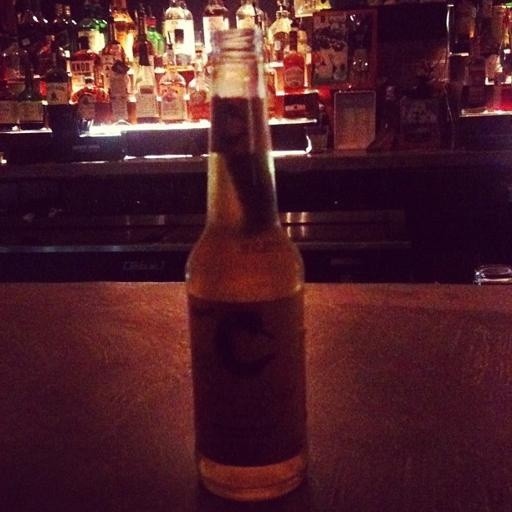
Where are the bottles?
[0,0,318,133]
[182,24,310,502]
[463,37,488,108]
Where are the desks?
[0,280,512,512]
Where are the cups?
[302,124,331,153]
